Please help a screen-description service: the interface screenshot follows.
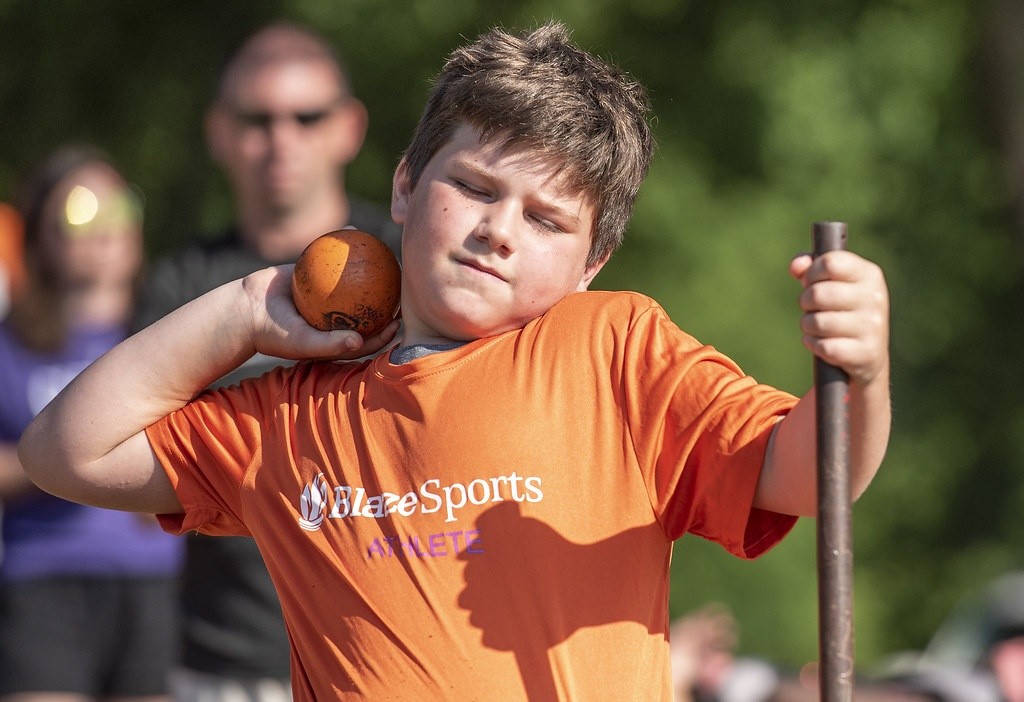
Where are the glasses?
[214,96,346,139]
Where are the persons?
[0,155,192,702]
[134,17,396,702]
[14,30,890,702]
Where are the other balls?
[289,228,402,344]
